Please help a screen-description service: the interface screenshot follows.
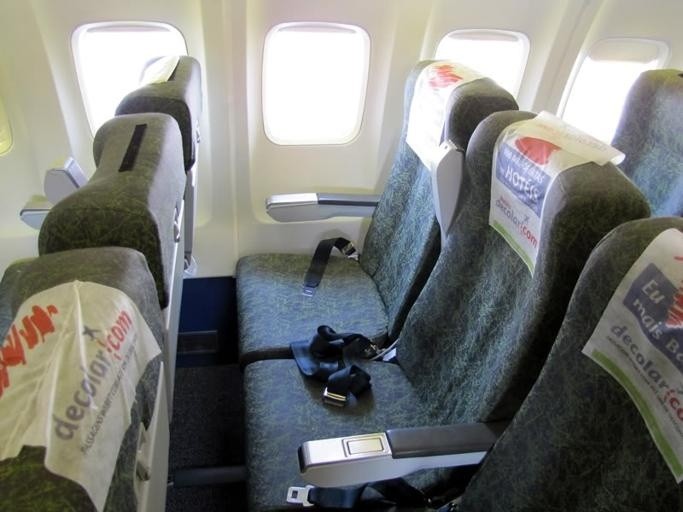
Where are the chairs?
[230,56,682,512]
[1,56,208,512]
